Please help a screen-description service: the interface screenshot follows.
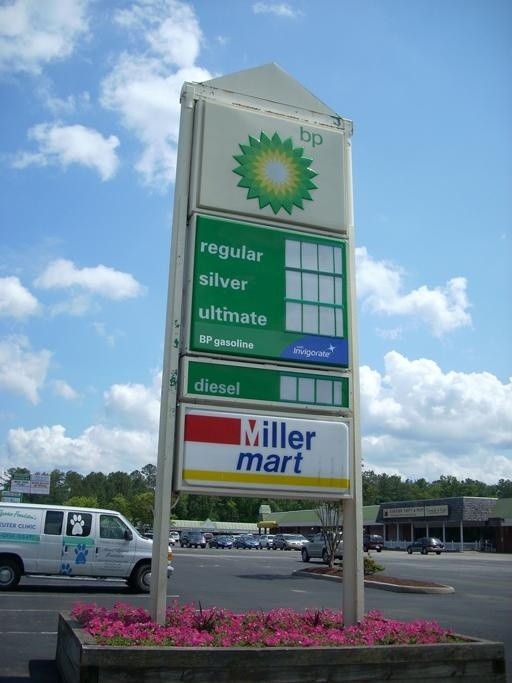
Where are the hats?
[407,536,445,555]
[363,534,384,552]
[301,533,343,562]
[144,529,310,551]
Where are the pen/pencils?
[0,501,175,593]
